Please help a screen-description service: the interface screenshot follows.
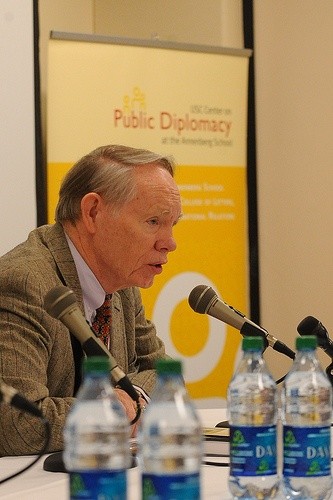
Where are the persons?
[0,144,188,459]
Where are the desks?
[0,410,236,498]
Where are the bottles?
[62,356,134,500]
[136,361,203,500]
[224,335,278,500]
[280,334,333,500]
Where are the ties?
[92,295,112,351]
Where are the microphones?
[297,316,333,357]
[188,285,296,361]
[0,380,45,421]
[44,286,141,401]
